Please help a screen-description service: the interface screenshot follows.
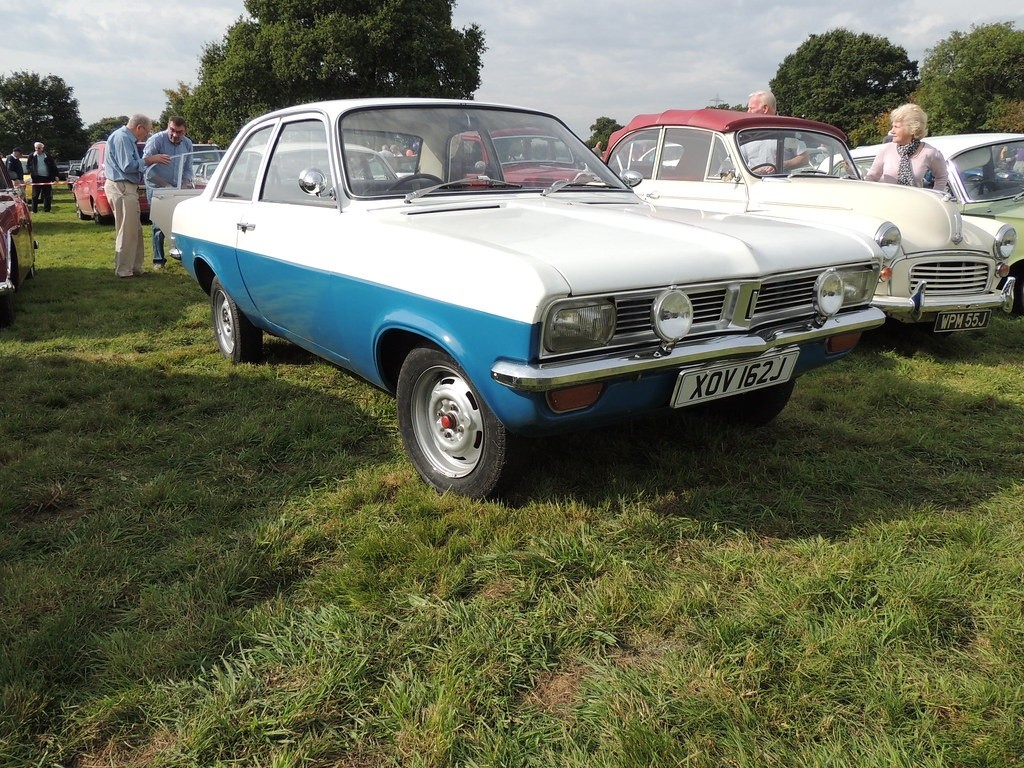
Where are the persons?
[863,103,948,191]
[26,142,59,214]
[719,90,809,176]
[104,113,193,278]
[592,141,604,160]
[5,147,27,203]
[377,144,414,158]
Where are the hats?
[13,147,24,152]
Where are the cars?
[0,157,34,204]
[55,132,1024,360]
[0,155,38,328]
[171,97,888,505]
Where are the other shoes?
[154,262,164,270]
[120,272,152,278]
